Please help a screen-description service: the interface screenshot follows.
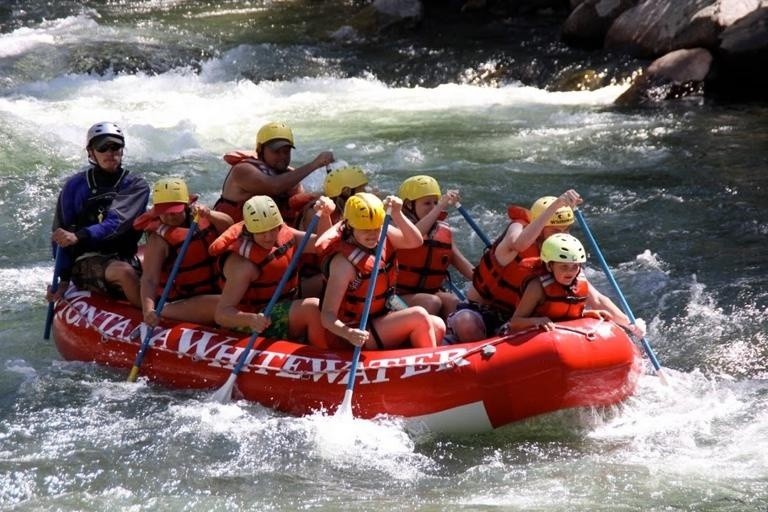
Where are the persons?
[451,189,647,343]
[509,232,613,335]
[213,120,335,230]
[295,165,369,234]
[134,178,235,327]
[315,192,447,351]
[396,175,475,315]
[45,121,150,310]
[207,195,337,351]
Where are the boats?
[50,241,641,431]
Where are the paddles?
[208,205,322,404]
[333,200,391,422]
[570,207,677,389]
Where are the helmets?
[323,166,368,198]
[400,175,440,202]
[539,233,586,263]
[152,177,189,206]
[242,195,284,235]
[86,121,125,147]
[529,196,574,227]
[256,121,296,152]
[342,191,387,230]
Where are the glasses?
[96,144,121,152]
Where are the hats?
[268,140,295,151]
[93,136,125,150]
[152,203,186,217]
